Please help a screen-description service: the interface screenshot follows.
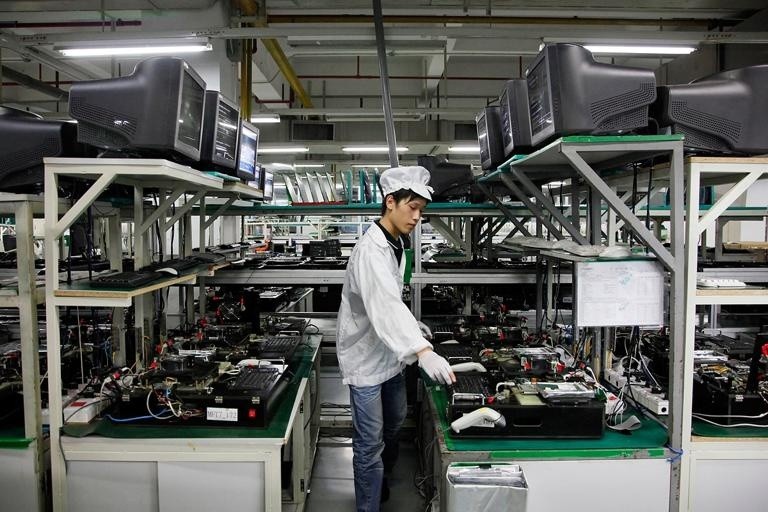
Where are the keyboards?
[137,243,241,273]
[504,237,605,256]
[696,278,747,287]
[90,271,161,289]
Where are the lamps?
[539,35,699,58]
[50,36,213,57]
[247,112,281,126]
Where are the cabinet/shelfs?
[0,133,768,512]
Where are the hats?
[379,165,434,202]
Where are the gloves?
[419,350,457,385]
[417,320,434,340]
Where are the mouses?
[600,246,633,259]
[155,266,179,276]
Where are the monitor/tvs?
[196,90,243,172]
[648,65,768,156]
[497,81,531,158]
[70,56,206,167]
[229,118,273,200]
[475,108,504,171]
[0,104,76,193]
[524,42,657,147]
[419,155,472,203]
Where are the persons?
[335,165,458,512]
